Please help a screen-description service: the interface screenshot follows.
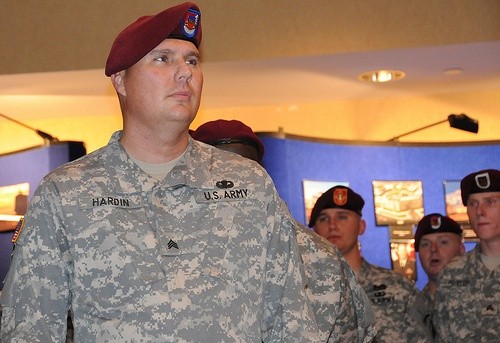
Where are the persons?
[414,213,465,300]
[308,186,436,343]
[192,120,377,343]
[432,169,500,343]
[0,0,321,343]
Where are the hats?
[192,118,265,164]
[307,185,364,228]
[104,2,203,77]
[414,213,462,251]
[459,169,500,206]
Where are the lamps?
[389,113,479,143]
